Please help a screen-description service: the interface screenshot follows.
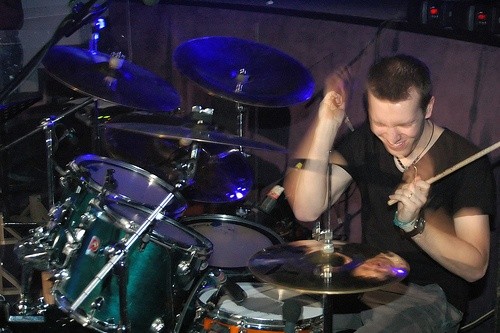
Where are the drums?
[192,283,325,333]
[177,214,285,302]
[53,193,214,333]
[56,154,187,221]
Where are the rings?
[408,193,413,199]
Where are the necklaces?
[395,118,434,183]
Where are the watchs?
[392,207,425,240]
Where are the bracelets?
[393,211,418,230]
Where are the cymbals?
[107,130,285,187]
[173,36,315,107]
[127,143,254,202]
[101,124,285,152]
[248,240,411,294]
[41,46,178,111]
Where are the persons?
[287,240,410,288]
[284,54,496,333]
